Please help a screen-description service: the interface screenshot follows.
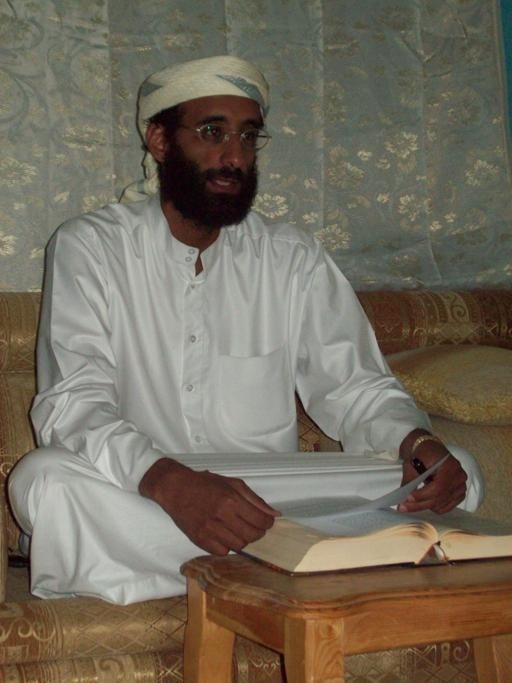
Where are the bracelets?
[411,434,441,453]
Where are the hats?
[136,55,269,141]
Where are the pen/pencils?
[411,459,434,484]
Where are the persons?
[7,55,484,605]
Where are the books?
[230,495,512,577]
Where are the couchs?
[2,288,512,682]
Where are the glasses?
[178,124,273,150]
